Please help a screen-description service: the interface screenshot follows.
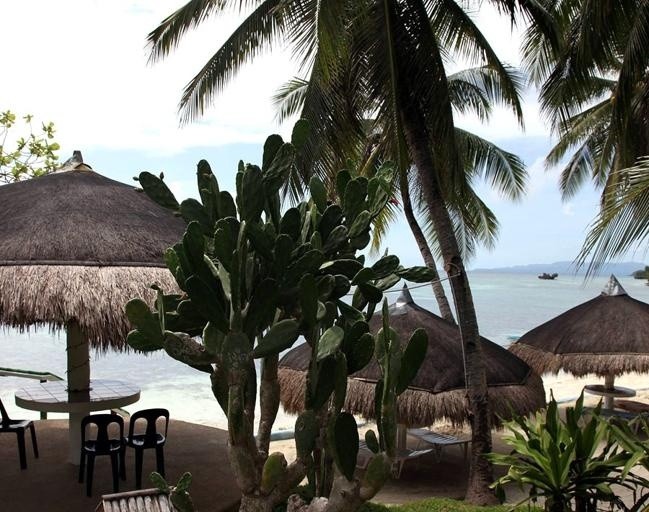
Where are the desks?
[584,385,636,409]
[17,379,141,466]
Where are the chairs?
[78,414,126,497]
[0,399,39,470]
[583,406,648,436]
[353,439,432,480]
[408,428,471,462]
[118,409,169,490]
[614,399,649,413]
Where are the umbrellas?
[0,151,186,468]
[507,273,648,409]
[277,281,550,468]
